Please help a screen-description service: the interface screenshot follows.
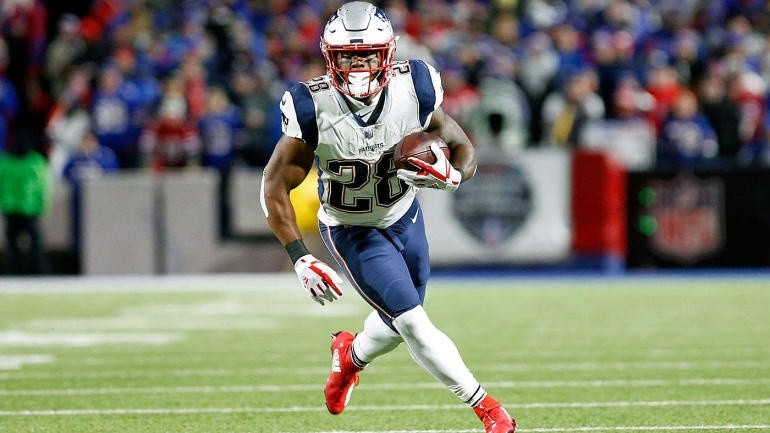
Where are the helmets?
[320,2,400,98]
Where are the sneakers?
[484,404,516,433]
[324,331,360,415]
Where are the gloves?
[294,254,343,306]
[397,142,461,192]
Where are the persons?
[260,1,517,433]
[0,0,770,274]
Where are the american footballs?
[393,132,451,171]
